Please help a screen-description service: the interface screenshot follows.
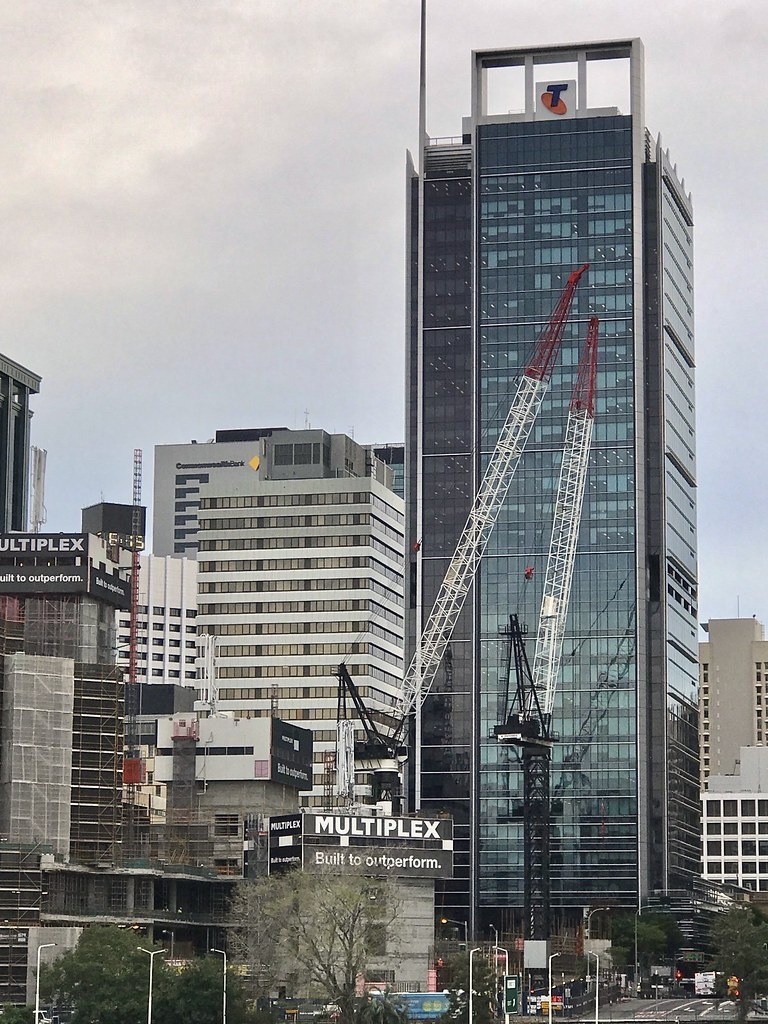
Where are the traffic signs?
[682,950,704,963]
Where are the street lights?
[588,950,600,1024]
[588,908,610,975]
[548,952,561,1024]
[492,946,511,1024]
[489,923,499,1012]
[469,948,483,1024]
[210,947,227,1024]
[635,904,671,999]
[442,918,469,953]
[137,945,169,1024]
[32,943,59,1024]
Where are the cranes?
[334,266,590,819]
[493,317,599,1024]
[118,448,146,872]
